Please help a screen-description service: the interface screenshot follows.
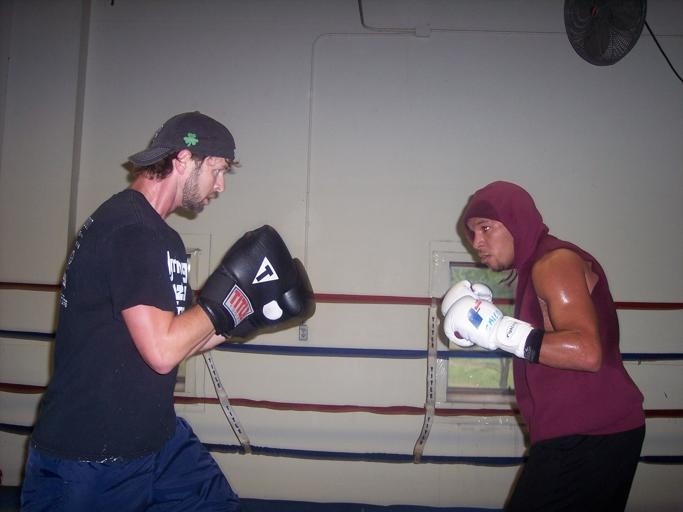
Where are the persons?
[440,181,646,511]
[18,109,316,511]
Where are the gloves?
[441,280,533,360]
[199,225,316,345]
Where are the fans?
[563,0,648,67]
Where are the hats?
[128,111,236,166]
